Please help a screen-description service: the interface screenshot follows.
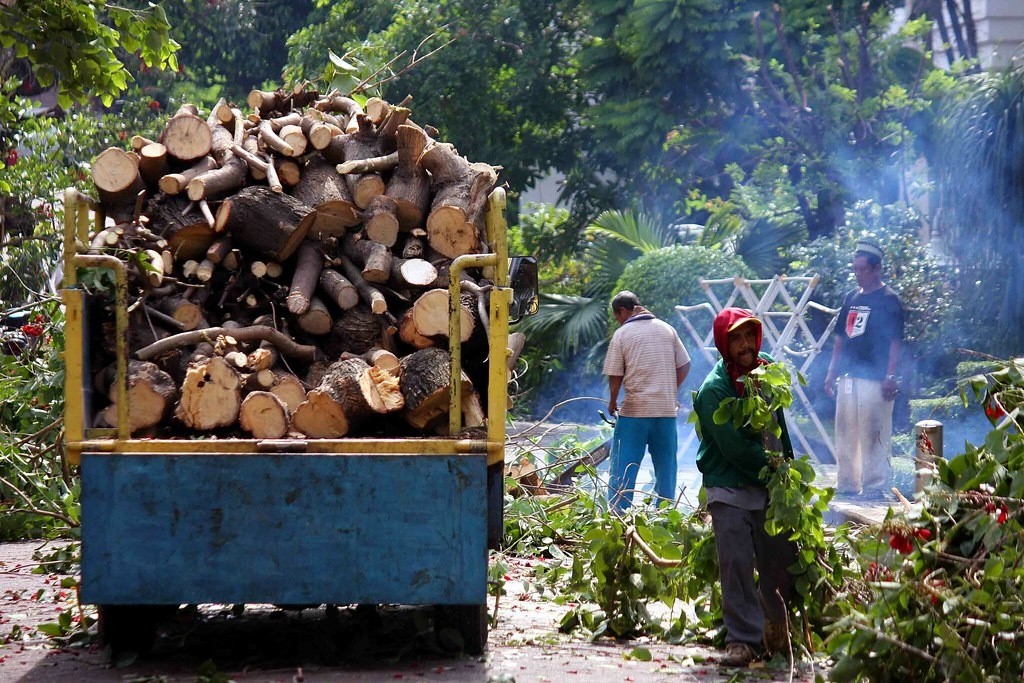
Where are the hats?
[728,316,762,332]
[855,241,885,260]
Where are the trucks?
[59,185,541,661]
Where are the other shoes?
[726,641,751,665]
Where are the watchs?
[886,374,897,382]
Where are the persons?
[601,290,692,516]
[822,239,904,500]
[693,307,800,664]
